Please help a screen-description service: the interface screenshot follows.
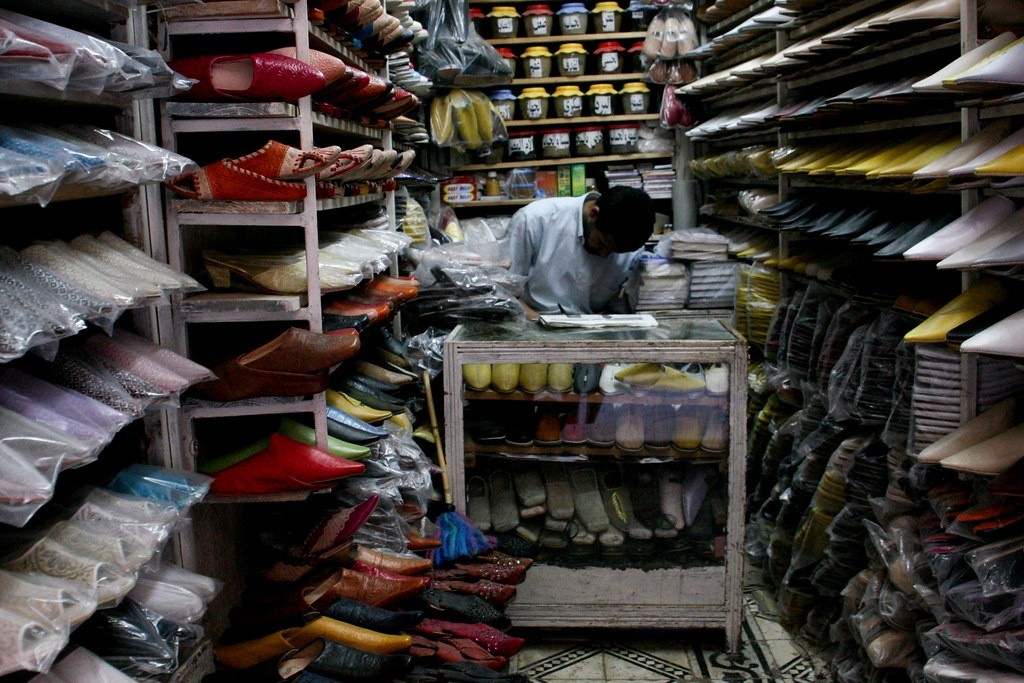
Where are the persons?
[494,185,656,322]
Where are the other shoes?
[0,0,1023,683]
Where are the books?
[627,232,743,310]
[605,164,676,199]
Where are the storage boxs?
[439,161,587,203]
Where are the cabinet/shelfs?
[130,1,406,569]
[442,312,748,659]
[1,0,214,683]
[431,30,673,204]
[692,0,1024,427]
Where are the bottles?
[485,171,500,196]
